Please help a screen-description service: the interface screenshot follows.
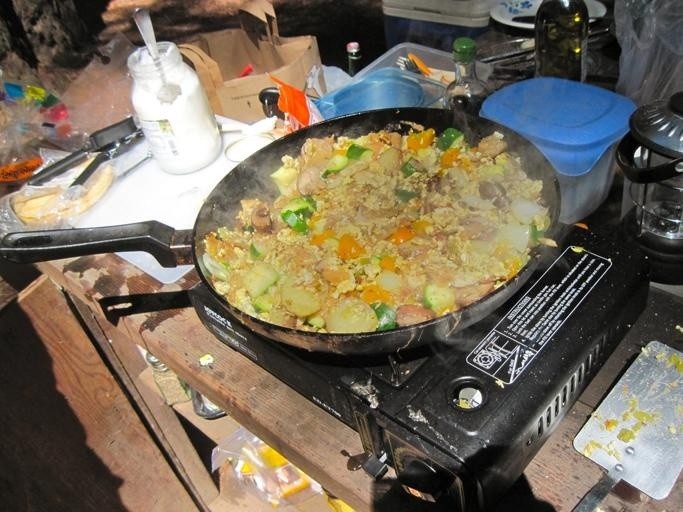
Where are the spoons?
[132,6,181,104]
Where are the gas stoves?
[184,223,653,512]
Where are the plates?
[490,0,607,32]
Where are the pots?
[0,106,562,358]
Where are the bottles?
[534,0,589,85]
[144,352,191,408]
[125,40,223,176]
[345,41,363,77]
[444,37,491,111]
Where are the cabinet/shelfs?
[0,253,683,512]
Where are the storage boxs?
[479,76,640,224]
[382,1,497,55]
